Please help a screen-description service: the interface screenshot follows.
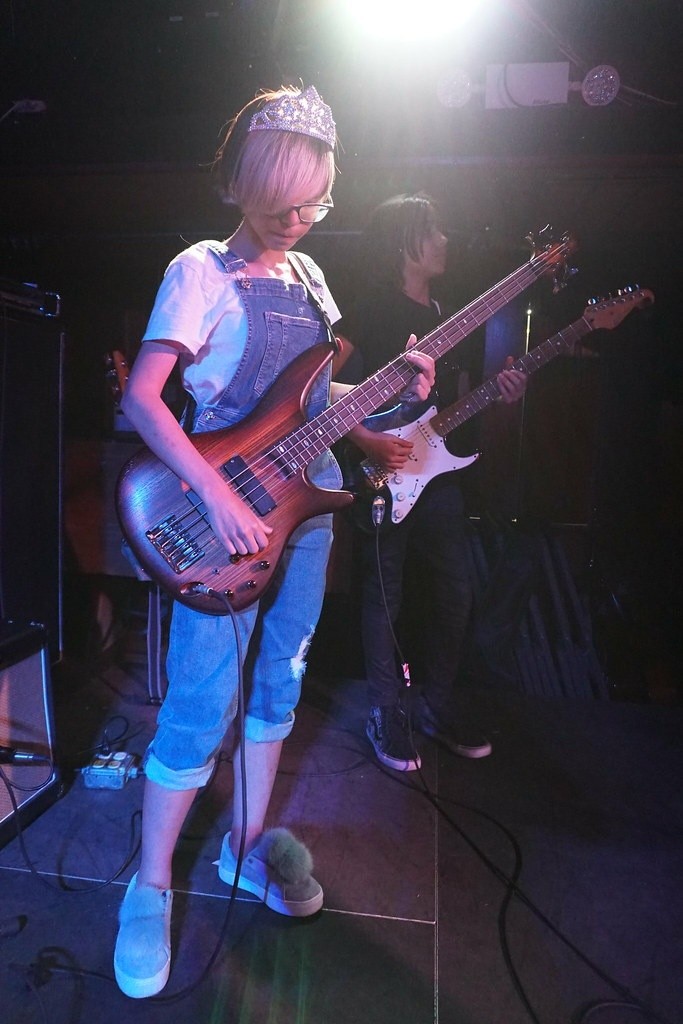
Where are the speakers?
[0,616,64,850]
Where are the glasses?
[267,195,334,223]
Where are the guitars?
[362,280,661,533]
[111,221,587,619]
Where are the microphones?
[0,746,48,765]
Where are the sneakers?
[218,827,323,918]
[411,690,491,757]
[113,870,174,999]
[365,705,422,771]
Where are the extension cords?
[83,750,138,790]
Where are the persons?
[87,583,117,669]
[114,83,436,998]
[342,195,529,773]
